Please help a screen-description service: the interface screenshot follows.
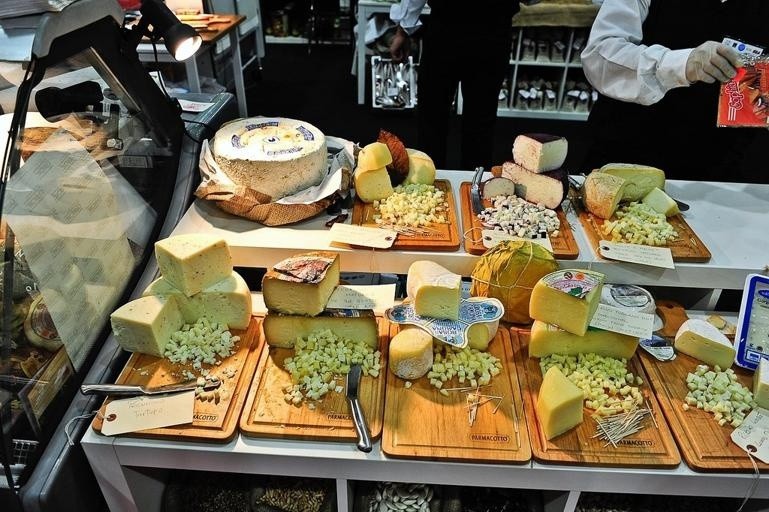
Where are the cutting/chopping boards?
[174,14,246,45]
[90,301,769,475]
[347,179,711,263]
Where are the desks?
[138,9,250,118]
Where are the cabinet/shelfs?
[456,0,601,121]
[79,163,769,511]
[1,1,241,511]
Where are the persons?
[573,0,769,186]
[390,2,539,171]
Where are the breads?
[537,365,584,441]
[261,251,380,351]
[110,234,252,359]
[674,319,736,371]
[481,133,569,210]
[582,162,680,220]
[752,357,769,410]
[388,260,501,380]
[528,268,640,360]
[354,142,436,204]
[212,117,329,202]
